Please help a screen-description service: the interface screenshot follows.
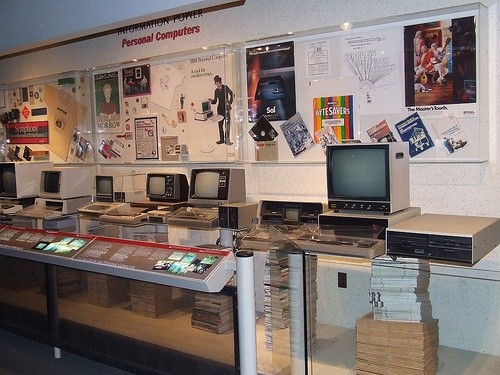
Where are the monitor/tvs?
[39,170,62,198]
[146,174,188,203]
[325,141,409,212]
[0,162,16,198]
[96,175,122,202]
[190,168,245,203]
[196,100,211,113]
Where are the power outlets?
[337,272,348,288]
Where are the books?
[129,279,174,319]
[355,310,439,375]
[191,293,234,334]
[87,272,121,307]
[40,266,79,297]
[263,250,319,356]
[369,257,433,322]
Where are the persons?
[124,78,131,94]
[414,30,449,94]
[141,75,148,92]
[208,74,234,145]
